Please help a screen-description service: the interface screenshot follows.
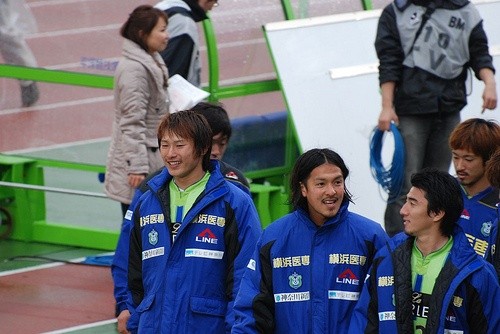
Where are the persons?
[0,0,39,107]
[374,0,497,238]
[103,5,174,220]
[345,169,500,334]
[154,0,219,86]
[112,109,262,334]
[193,102,250,189]
[447,119,500,268]
[230,148,390,334]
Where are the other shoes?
[20,81,39,108]
